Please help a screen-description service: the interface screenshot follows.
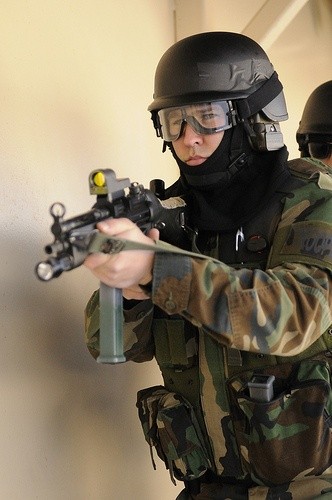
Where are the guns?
[34,165,195,365]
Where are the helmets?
[297,79,332,147]
[147,33,289,122]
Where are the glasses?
[158,99,235,142]
[302,138,332,159]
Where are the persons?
[82,32,332,500]
[296,80,332,169]
